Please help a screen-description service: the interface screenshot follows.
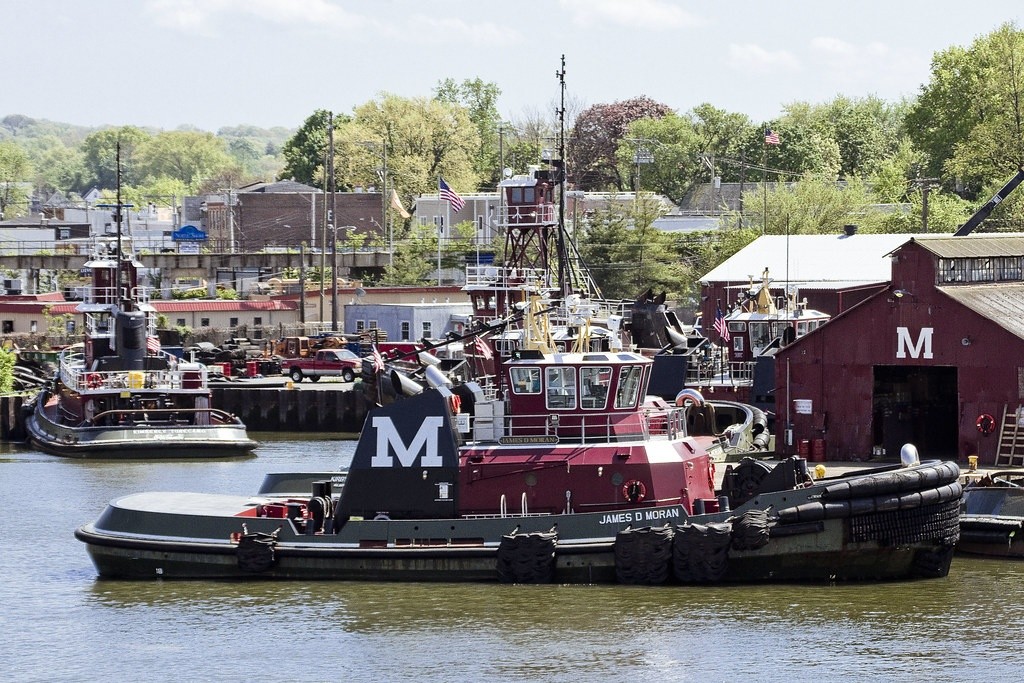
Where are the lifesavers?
[707,466,714,487]
[623,480,646,503]
[449,394,461,414]
[88,374,102,389]
[975,413,996,433]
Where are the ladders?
[994,404,1024,468]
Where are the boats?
[22,141,257,454]
[75,50,960,585]
[957,486,1024,559]
[683,266,832,408]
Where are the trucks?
[340,342,435,362]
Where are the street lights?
[367,146,387,250]
[326,223,357,332]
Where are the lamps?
[893,290,915,299]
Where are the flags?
[765,128,780,144]
[147,337,158,352]
[475,335,493,361]
[391,187,410,219]
[372,344,384,373]
[713,304,731,342]
[440,177,466,212]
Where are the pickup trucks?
[279,349,362,382]
